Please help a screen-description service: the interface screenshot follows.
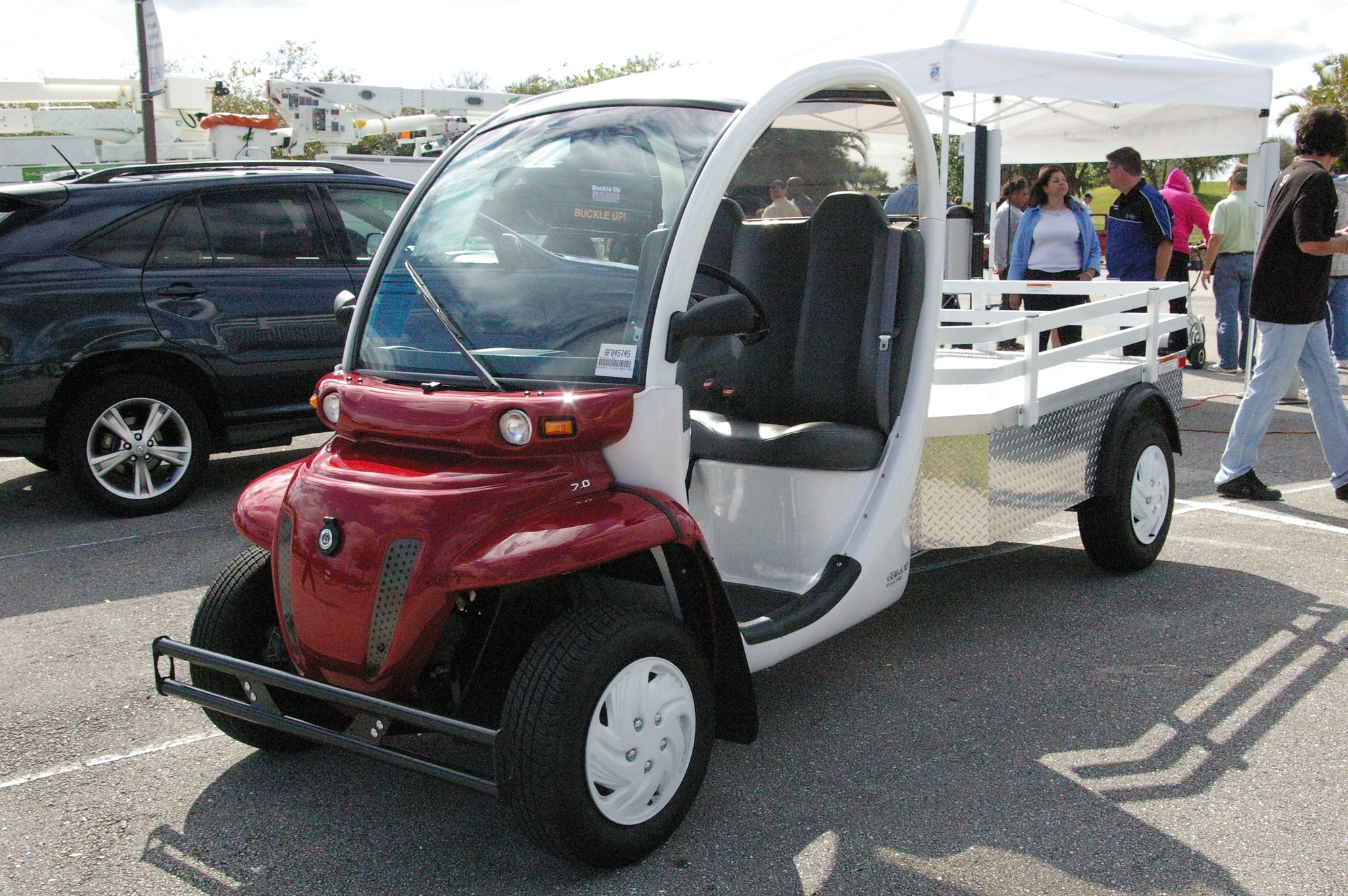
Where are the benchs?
[692,191,906,471]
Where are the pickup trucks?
[139,47,1208,867]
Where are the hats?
[1085,193,1093,198]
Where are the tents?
[770,0,1272,398]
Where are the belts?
[1217,252,1254,258]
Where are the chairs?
[287,241,288,242]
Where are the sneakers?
[1333,355,1348,369]
[1335,483,1348,499]
[1216,468,1282,500]
[1207,363,1237,373]
[1243,367,1254,373]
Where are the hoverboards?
[1156,241,1208,370]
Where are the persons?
[763,148,1348,374]
[1215,104,1348,499]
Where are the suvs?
[0,148,708,520]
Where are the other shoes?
[997,341,1024,351]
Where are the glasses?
[1106,166,1127,173]
